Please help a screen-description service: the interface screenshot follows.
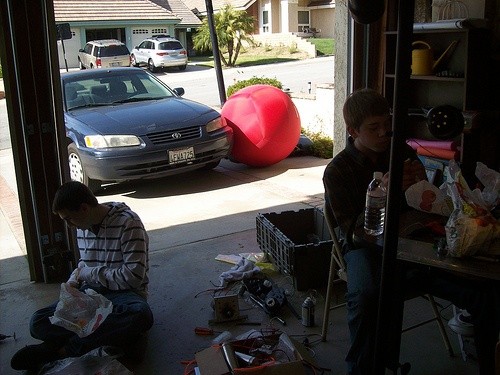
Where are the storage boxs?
[194,333,321,375]
[255,207,334,291]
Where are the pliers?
[194,328,225,337]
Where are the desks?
[383,238,500,375]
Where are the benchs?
[77,85,109,102]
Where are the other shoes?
[9,342,58,371]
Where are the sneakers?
[447,314,478,336]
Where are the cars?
[60,66,233,193]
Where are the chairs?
[66,87,84,106]
[110,78,127,98]
[321,203,453,354]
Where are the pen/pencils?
[237,322,261,326]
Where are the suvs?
[131,37,189,72]
[77,39,132,70]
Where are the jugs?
[411,41,456,75]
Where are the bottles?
[364,172,387,236]
[302,297,315,327]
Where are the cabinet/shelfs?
[384,28,490,158]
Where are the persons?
[10,181,153,371]
[322,88,429,375]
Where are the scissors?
[208,318,230,326]
